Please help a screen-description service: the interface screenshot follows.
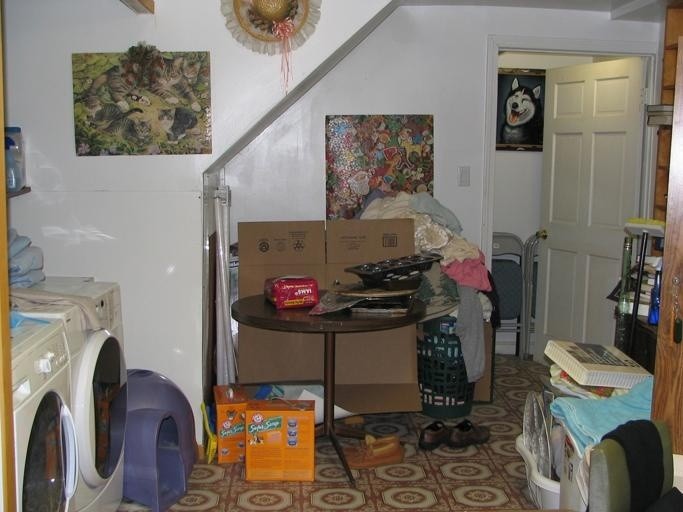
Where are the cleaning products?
[648,257,663,325]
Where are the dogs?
[501,76,545,143]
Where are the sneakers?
[417,419,451,451]
[448,418,491,451]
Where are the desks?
[232,290,424,488]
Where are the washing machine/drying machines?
[20,277,128,512]
[11,319,77,512]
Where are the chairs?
[590,420,675,512]
[492,226,540,360]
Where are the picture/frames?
[497,66,546,152]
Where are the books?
[604,255,662,316]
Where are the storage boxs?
[214,385,247,464]
[557,420,592,512]
[245,401,316,482]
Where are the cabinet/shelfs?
[614,308,661,375]
